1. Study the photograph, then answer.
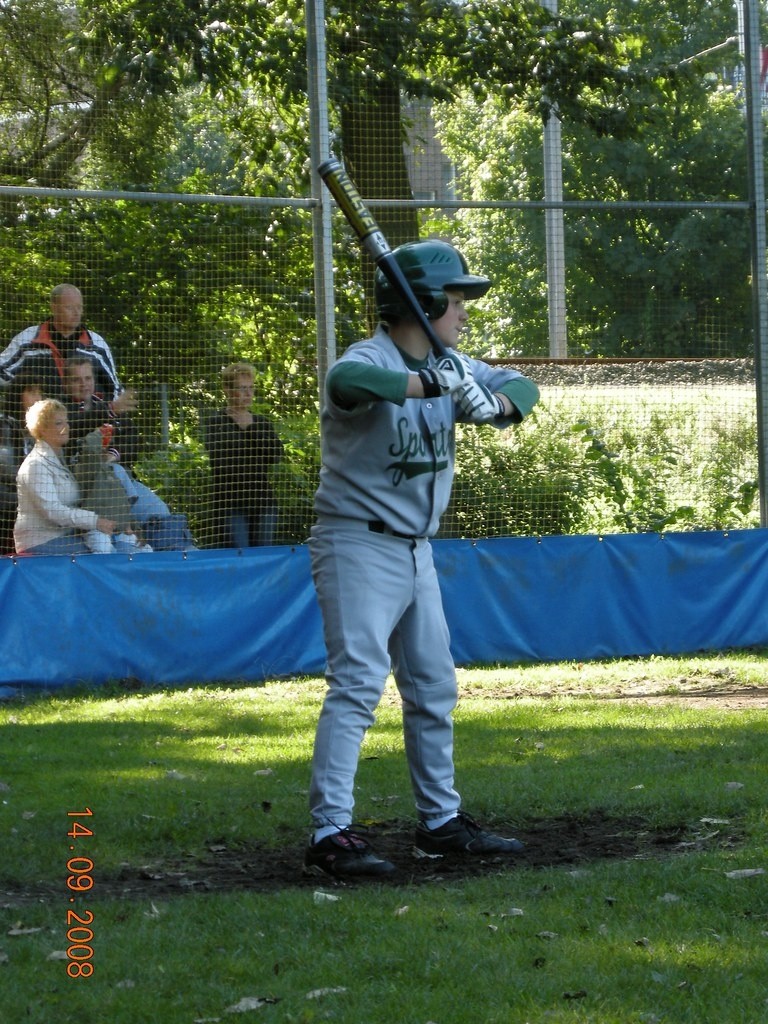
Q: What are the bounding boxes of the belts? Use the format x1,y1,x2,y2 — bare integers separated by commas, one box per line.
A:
368,519,417,540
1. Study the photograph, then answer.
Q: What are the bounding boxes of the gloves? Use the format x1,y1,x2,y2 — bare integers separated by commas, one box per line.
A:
419,356,474,398
458,381,505,420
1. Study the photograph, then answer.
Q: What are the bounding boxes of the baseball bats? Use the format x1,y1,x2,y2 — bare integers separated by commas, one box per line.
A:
316,154,454,364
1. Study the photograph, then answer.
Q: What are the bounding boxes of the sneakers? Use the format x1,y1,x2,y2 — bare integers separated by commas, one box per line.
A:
414,815,522,866
304,822,397,885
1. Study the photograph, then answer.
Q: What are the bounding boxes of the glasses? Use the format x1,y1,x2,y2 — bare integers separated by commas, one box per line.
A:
236,385,255,391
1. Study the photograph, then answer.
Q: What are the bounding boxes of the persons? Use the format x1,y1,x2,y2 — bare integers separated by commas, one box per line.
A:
0,283,125,444
9,373,47,464
64,356,138,463
203,363,285,548
13,399,153,553
302,240,540,884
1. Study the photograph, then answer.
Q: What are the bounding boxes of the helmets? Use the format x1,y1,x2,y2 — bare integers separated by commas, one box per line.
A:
376,241,491,321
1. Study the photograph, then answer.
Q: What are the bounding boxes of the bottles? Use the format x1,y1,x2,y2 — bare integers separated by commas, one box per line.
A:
99,423,113,450
